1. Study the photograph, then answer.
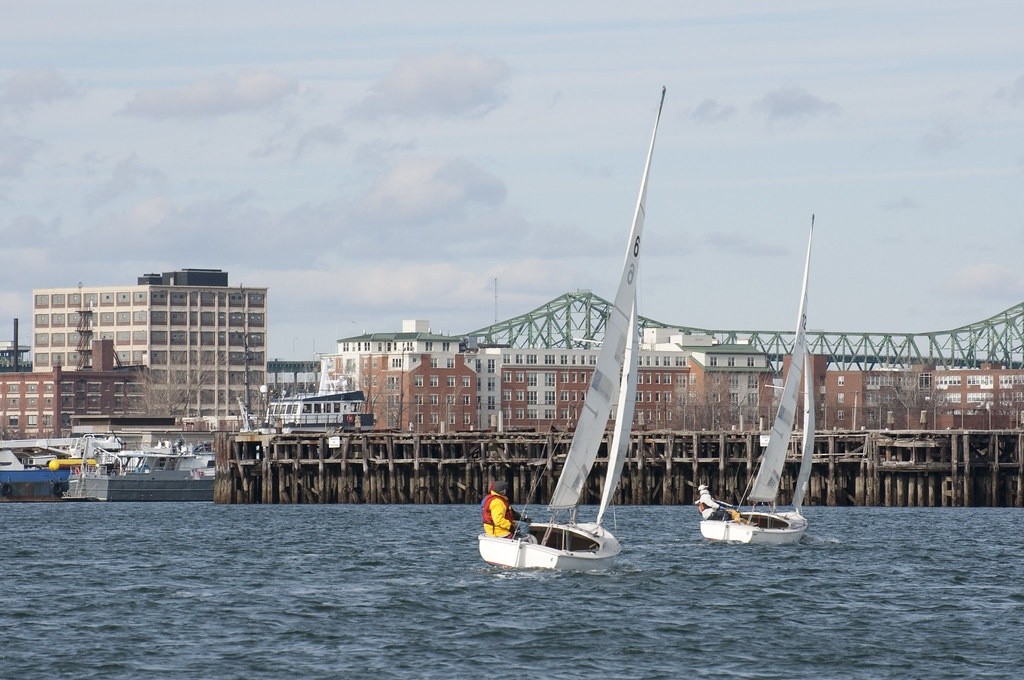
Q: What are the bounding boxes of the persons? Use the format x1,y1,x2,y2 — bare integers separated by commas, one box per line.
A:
481,481,531,540
694,485,733,521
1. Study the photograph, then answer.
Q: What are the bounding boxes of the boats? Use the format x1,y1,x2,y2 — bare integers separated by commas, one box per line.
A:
254,388,376,433
67,440,220,505
0,428,124,503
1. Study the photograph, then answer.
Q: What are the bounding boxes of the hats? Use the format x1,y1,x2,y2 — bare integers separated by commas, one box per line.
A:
698,485,708,491
695,494,711,504
493,481,509,491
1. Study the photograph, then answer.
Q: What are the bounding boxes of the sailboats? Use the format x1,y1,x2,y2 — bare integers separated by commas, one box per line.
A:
474,85,670,570
697,212,819,544
